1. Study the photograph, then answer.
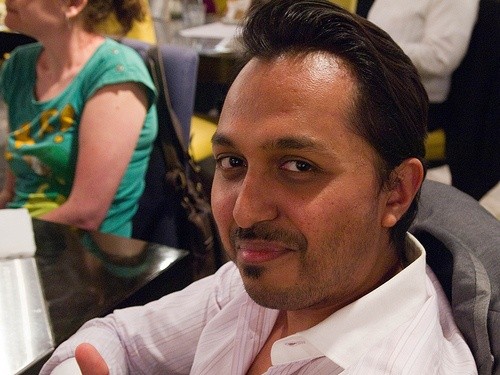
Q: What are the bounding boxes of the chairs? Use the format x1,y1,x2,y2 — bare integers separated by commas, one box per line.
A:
119,39,198,294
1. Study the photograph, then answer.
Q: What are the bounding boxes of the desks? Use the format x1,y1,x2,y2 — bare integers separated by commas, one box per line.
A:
156,15,250,119
0,217,191,375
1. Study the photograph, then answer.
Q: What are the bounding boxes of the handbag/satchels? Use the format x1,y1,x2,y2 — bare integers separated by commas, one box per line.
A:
148,46,228,283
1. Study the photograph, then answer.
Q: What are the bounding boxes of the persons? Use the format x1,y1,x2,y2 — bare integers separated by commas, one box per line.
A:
0,0,158,237
38,0,480,375
